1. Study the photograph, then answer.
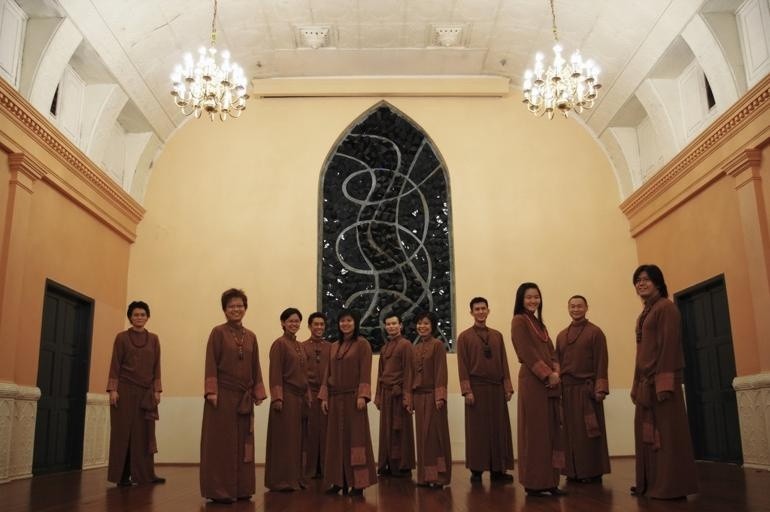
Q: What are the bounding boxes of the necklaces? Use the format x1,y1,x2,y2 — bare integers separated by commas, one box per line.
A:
566,319,589,345
309,337,324,364
128,326,150,349
473,325,493,360
336,338,354,361
227,320,246,361
635,294,667,345
524,312,549,344
383,338,398,360
295,339,306,367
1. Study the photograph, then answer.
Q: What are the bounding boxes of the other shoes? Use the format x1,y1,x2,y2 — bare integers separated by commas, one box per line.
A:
205,497,237,504
271,487,293,492
433,482,443,488
349,486,363,496
238,495,253,501
416,482,431,488
568,476,603,482
490,471,514,483
470,474,483,489
392,469,412,479
631,486,637,492
325,484,341,495
524,486,569,496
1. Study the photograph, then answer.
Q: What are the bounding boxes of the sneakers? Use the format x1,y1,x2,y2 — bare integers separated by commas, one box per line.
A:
116,475,166,487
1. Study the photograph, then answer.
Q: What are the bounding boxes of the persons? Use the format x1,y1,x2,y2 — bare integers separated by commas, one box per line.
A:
372,312,414,482
555,295,612,486
456,296,514,483
302,311,334,480
198,287,267,506
317,308,378,498
510,282,570,498
264,307,313,494
106,299,166,488
630,264,692,501
402,311,453,490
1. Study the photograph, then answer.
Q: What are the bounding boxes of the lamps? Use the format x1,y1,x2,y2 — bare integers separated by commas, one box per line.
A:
520,0,602,120
166,1,255,121
